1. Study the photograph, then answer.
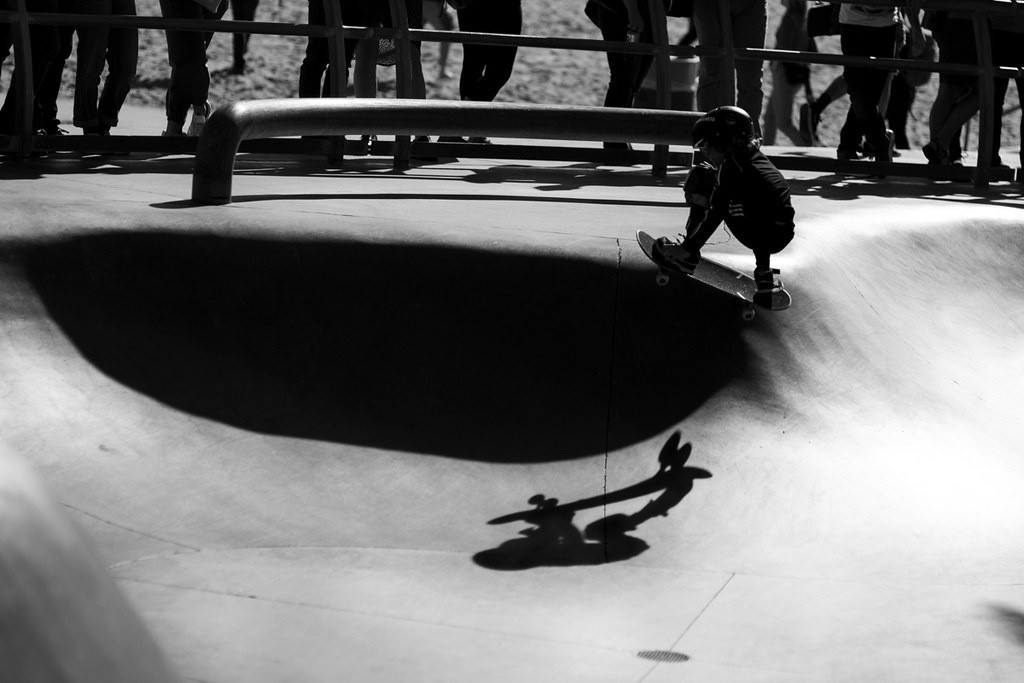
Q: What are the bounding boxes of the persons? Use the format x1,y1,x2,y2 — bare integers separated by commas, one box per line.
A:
0,0,1024,194
656,106,795,293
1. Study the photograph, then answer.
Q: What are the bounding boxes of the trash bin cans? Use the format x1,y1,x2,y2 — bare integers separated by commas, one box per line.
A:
632,54,701,114
